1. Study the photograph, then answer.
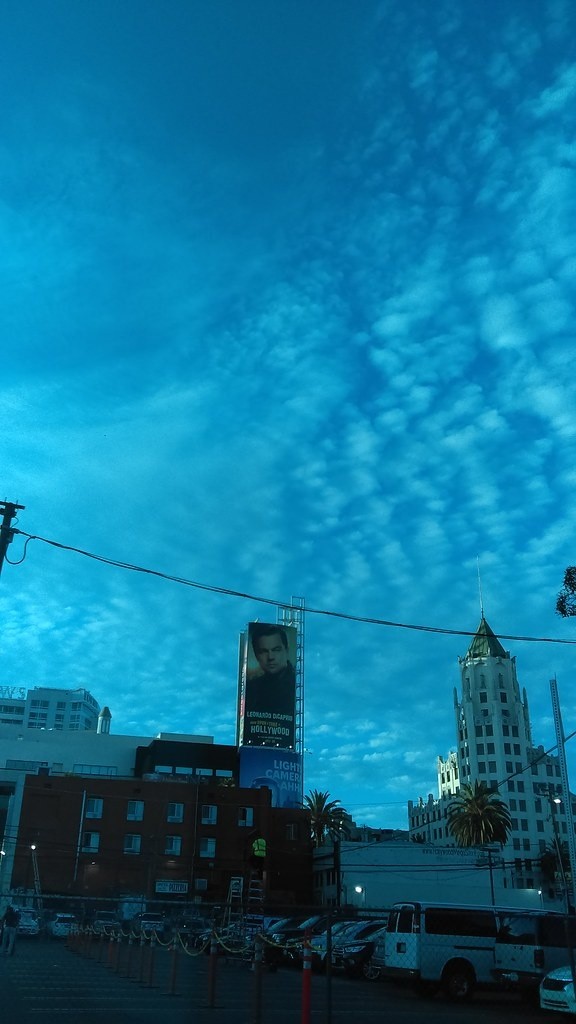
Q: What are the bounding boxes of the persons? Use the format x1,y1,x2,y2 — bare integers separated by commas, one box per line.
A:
0,905,17,957
243,622,299,749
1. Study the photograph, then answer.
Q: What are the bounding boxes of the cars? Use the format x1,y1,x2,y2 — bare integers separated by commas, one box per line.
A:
9,892,576,1015
